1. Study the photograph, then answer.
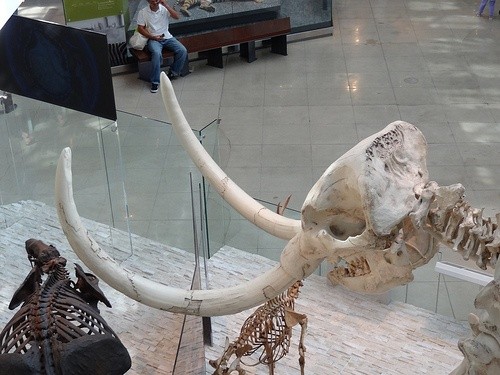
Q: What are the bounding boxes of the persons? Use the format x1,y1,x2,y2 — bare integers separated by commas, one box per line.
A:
136,0,187,93
475,0,497,20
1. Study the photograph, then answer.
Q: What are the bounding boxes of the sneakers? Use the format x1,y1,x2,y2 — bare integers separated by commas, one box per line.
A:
168,72,174,80
151,83,158,92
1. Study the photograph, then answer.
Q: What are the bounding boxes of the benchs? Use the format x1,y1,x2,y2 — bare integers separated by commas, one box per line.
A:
128,15,292,80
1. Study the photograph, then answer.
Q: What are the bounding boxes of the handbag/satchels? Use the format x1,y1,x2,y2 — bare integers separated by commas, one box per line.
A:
128,31,148,50
